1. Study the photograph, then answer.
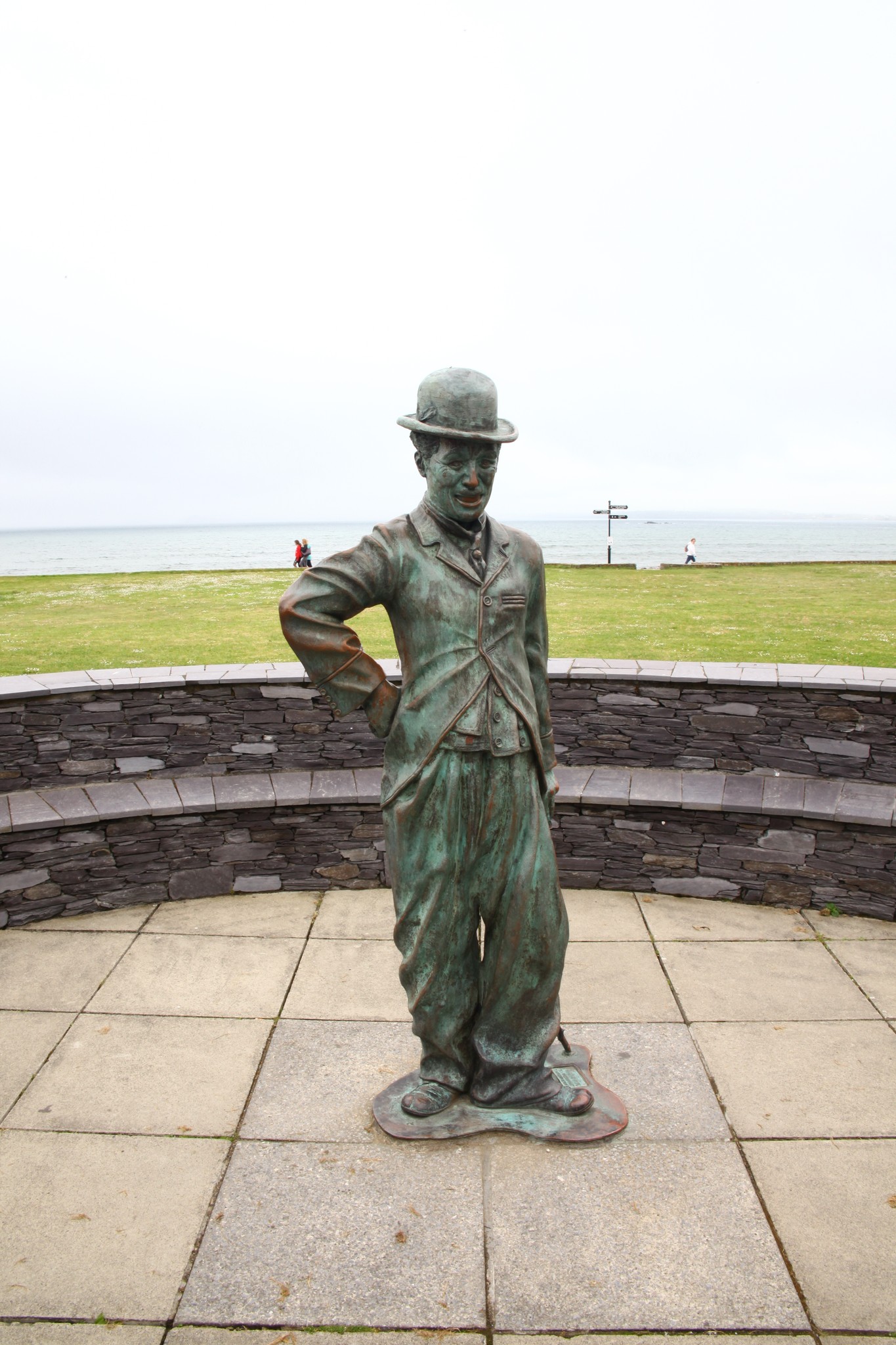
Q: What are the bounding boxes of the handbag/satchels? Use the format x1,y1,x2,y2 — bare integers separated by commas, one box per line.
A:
685,545,689,552
301,548,311,556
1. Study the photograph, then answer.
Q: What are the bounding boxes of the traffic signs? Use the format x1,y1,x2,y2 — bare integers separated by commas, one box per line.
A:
608,515,628,519
593,510,610,514
609,505,628,509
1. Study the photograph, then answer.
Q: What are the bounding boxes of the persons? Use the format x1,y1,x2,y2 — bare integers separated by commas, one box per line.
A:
276,365,600,1118
299,538,313,567
293,540,304,567
684,538,697,564
